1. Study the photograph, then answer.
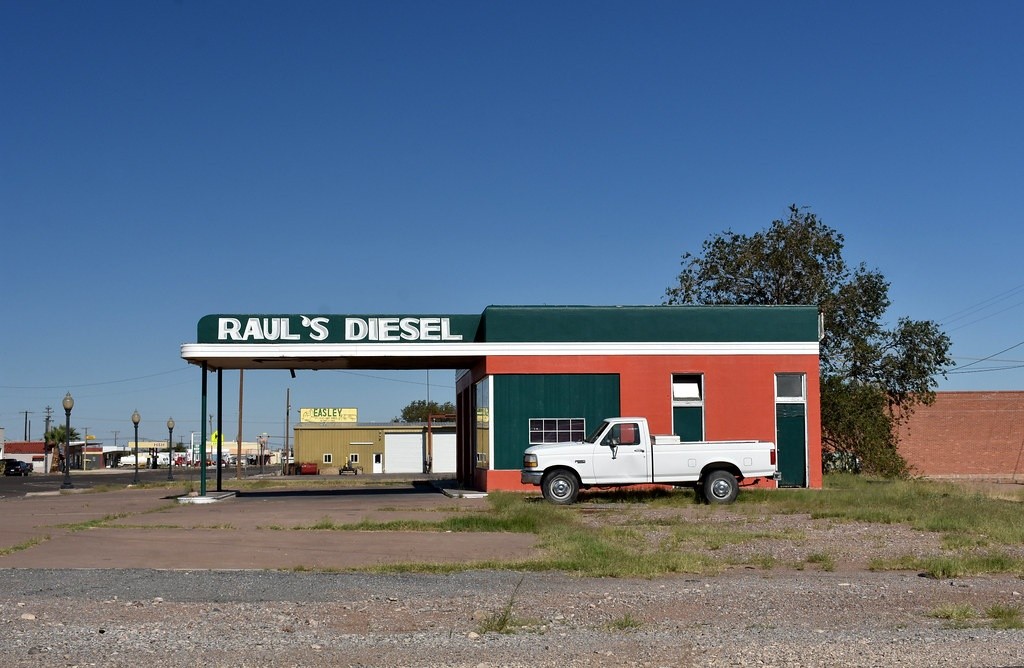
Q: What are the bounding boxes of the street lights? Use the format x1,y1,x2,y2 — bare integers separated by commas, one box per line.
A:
131,408,141,485
167,416,175,482
60,391,75,489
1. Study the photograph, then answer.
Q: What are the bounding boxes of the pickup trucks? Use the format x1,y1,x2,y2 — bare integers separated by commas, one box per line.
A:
521,417,782,507
0,459,33,472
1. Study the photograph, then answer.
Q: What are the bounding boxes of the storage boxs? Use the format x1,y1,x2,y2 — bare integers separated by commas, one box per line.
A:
650,434,680,445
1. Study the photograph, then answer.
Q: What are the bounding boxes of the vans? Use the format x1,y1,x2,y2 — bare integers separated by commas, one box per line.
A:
4,461,27,477
